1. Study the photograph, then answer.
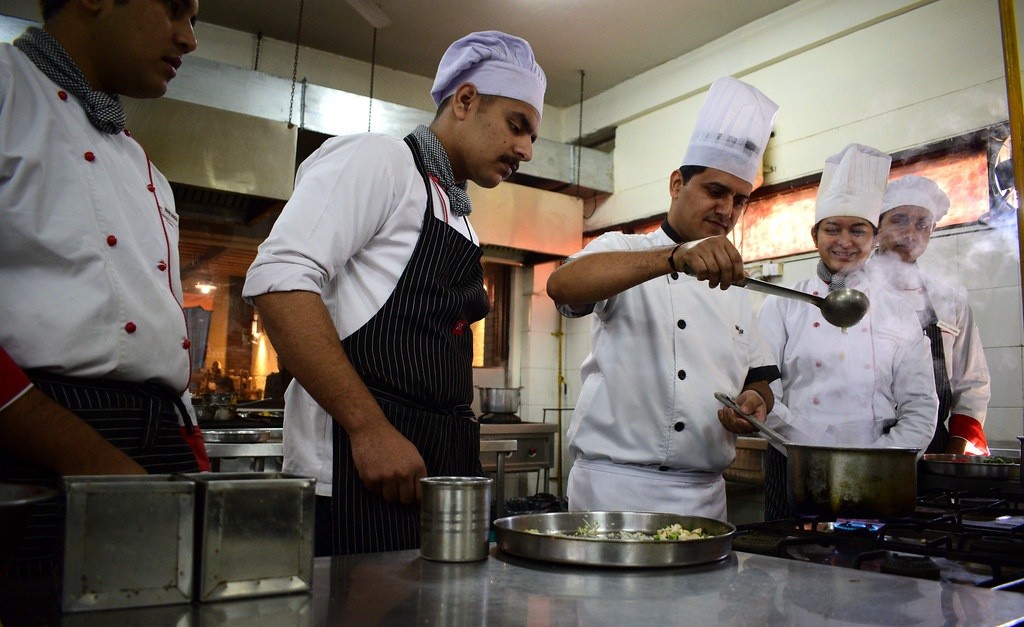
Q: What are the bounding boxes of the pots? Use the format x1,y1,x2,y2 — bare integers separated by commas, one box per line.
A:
193,397,273,422
918,454,1020,489
474,385,524,413
714,393,922,518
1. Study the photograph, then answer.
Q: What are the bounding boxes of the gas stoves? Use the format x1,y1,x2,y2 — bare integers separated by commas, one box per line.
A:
731,436,1024,591
478,413,558,471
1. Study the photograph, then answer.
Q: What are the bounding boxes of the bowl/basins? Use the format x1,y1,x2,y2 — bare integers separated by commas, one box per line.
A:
202,393,235,403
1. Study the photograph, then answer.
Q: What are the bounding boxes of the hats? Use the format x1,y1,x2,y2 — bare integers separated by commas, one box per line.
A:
880,175,951,223
429,30,547,120
814,143,892,228
681,77,779,192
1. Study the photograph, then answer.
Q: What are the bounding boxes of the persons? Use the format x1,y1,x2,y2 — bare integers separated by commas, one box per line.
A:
243,27,548,557
543,131,782,525
1,2,215,612
862,174,993,456
756,177,939,519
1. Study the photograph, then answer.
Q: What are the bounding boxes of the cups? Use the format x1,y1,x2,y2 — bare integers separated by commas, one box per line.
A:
419,477,493,561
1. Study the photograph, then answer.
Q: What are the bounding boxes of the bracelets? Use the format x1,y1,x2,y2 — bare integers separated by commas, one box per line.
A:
668,243,687,273
